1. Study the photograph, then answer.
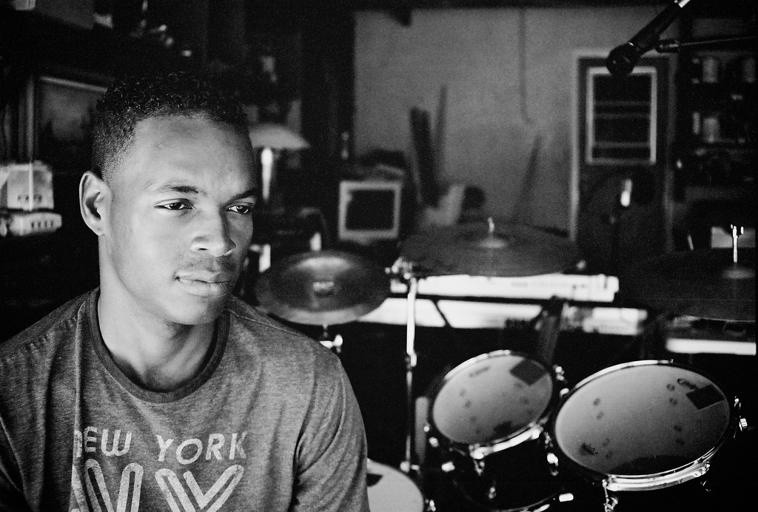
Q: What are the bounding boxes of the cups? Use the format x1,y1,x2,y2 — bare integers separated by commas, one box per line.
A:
689,52,723,145
739,55,758,83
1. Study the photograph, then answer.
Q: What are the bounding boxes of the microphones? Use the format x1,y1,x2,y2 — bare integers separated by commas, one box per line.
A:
607,0,691,80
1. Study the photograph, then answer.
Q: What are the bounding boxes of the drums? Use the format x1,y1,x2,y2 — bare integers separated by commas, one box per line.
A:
366,457,423,512
423,348,572,512
538,358,749,512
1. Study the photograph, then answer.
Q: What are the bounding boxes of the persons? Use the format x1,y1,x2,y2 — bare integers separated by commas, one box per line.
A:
0,72,369,511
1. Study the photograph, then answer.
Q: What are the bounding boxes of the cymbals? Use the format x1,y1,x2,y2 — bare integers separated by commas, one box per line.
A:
400,220,579,276
252,251,390,327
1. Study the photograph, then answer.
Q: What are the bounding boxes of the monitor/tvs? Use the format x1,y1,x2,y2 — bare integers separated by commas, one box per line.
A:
337,180,402,242
